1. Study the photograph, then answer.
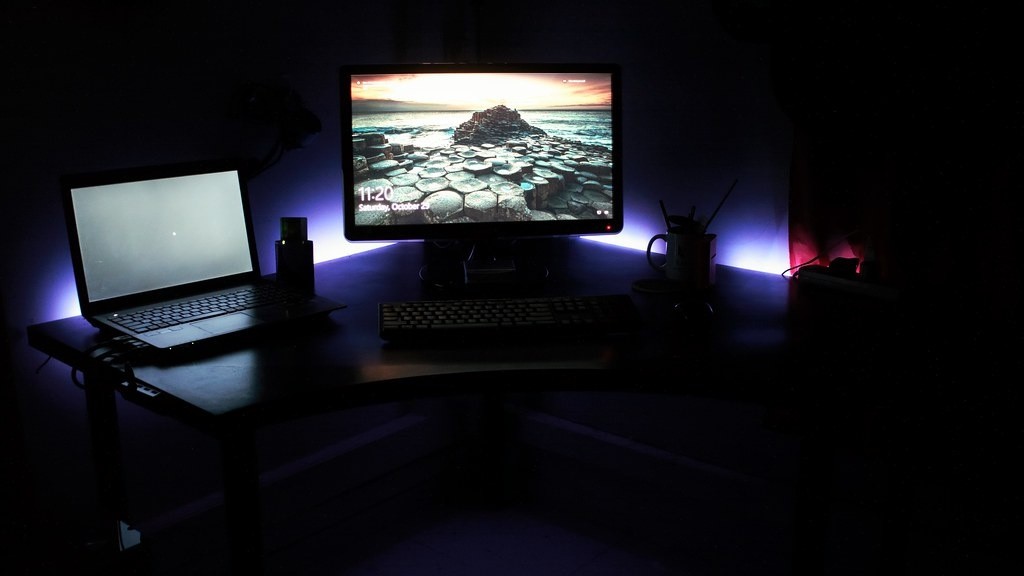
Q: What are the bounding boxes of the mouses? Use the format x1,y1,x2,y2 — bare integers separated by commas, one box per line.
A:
673,300,714,321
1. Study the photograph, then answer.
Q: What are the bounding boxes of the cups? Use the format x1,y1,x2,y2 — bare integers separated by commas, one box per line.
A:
647,228,716,288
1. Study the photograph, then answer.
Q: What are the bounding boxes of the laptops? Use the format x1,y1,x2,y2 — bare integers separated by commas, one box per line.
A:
56,158,347,359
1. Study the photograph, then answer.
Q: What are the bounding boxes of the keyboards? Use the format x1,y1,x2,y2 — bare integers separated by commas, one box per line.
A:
379,294,643,345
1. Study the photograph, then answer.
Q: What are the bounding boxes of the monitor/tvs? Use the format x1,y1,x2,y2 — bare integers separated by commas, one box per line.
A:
337,63,623,299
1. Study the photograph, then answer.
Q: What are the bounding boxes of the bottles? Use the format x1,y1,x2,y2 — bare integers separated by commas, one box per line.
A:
275,218,315,294
